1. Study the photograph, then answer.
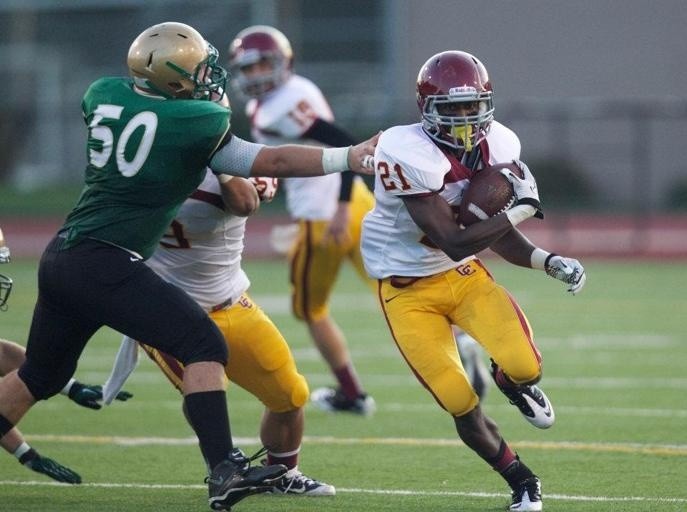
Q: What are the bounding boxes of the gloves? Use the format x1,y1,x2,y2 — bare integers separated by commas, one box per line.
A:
17,446,82,483
500,159,544,220
68,380,133,409
544,253,587,296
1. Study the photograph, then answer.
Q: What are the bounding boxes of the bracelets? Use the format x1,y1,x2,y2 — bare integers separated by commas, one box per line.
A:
505,205,537,223
217,173,238,183
528,247,549,270
320,145,351,176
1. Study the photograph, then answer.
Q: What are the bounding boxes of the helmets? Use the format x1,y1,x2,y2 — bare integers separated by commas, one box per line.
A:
227,25,292,102
416,50,496,152
0,229,14,313
127,22,227,104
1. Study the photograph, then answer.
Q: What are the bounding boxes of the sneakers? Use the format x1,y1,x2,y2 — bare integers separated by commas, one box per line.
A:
311,388,376,418
488,357,554,430
509,476,542,512
260,460,336,496
455,332,485,404
203,446,289,511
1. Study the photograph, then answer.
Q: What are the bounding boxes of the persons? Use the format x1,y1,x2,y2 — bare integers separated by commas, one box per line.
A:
1,228,135,483
360,50,587,511
227,26,492,419
141,79,340,499
1,22,384,510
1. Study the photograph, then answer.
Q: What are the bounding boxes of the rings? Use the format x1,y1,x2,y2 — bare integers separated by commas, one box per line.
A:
360,156,370,170
368,157,373,169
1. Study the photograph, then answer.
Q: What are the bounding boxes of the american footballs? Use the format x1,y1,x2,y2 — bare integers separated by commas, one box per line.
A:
459,162,525,226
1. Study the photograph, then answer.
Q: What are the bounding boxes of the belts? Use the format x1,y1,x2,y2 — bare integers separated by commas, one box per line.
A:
389,275,421,288
